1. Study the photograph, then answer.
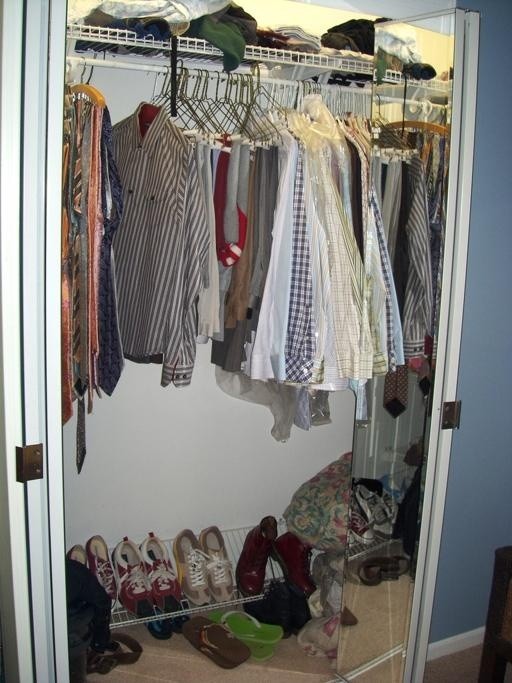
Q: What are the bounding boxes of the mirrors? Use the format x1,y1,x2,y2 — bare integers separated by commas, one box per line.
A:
342,6,459,681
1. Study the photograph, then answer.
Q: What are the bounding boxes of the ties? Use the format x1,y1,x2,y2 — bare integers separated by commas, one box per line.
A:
61,97,124,476
383,130,450,420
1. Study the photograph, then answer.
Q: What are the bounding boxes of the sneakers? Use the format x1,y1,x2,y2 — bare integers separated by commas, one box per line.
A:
355,473,419,538
350,510,376,546
114,538,154,616
142,536,182,606
88,535,119,610
68,543,86,565
173,528,211,606
200,526,234,603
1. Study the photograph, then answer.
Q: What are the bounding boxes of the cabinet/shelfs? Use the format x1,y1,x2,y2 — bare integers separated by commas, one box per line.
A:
372,94,452,159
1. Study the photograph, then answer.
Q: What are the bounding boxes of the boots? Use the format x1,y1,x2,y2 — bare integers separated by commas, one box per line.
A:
234,516,277,597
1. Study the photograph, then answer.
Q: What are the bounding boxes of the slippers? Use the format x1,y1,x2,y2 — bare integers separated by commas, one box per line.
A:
182,609,283,670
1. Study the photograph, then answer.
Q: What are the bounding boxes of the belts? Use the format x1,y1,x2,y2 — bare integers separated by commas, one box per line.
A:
357,555,411,588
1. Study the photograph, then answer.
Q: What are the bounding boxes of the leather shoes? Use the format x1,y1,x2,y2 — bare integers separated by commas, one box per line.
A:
242,582,313,638
146,602,183,641
270,532,317,600
